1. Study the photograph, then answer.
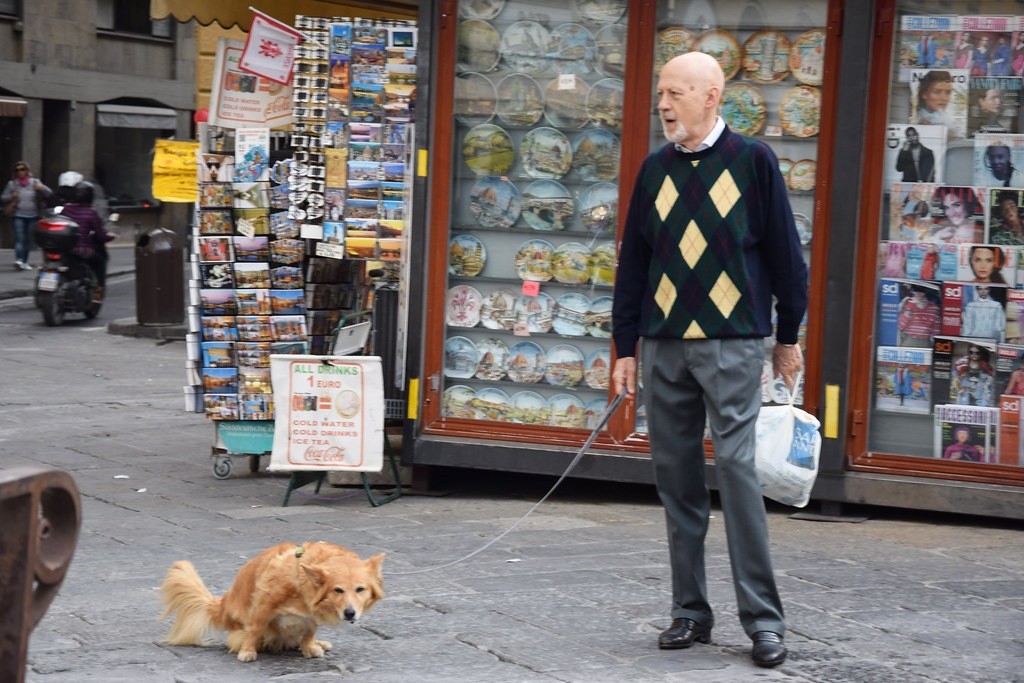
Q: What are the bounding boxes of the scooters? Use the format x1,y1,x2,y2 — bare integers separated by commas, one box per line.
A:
35,184,119,326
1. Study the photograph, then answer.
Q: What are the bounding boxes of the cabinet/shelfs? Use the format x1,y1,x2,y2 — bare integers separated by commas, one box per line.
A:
419,0,844,460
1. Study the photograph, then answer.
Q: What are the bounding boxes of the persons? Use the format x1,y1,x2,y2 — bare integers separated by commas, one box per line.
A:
1011,32,1024,77
991,190,1024,245
45,170,116,304
971,35,990,76
894,365,912,406
967,82,1007,139
962,286,1005,341
917,32,934,67
958,364,994,406
895,127,934,183
982,141,1023,189
914,71,955,137
954,31,973,69
898,286,940,347
991,36,1011,76
931,187,984,243
610,52,807,667
969,246,1005,284
919,243,940,279
956,344,993,376
0,161,53,269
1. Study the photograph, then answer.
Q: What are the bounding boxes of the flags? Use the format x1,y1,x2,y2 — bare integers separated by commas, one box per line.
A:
238,13,299,87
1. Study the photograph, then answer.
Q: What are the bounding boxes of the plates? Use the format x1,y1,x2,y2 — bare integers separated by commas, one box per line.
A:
792,213,813,246
447,285,483,327
778,86,822,138
515,292,555,334
586,296,614,339
777,158,817,190
460,124,621,181
480,287,518,331
552,293,590,337
454,72,625,132
652,25,827,84
442,385,651,440
468,176,619,238
721,82,767,135
570,0,627,28
759,359,805,405
443,335,612,391
457,20,627,78
456,0,507,20
447,235,487,277
513,238,617,287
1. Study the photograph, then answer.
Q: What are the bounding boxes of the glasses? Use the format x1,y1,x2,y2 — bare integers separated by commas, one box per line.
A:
15,168,27,171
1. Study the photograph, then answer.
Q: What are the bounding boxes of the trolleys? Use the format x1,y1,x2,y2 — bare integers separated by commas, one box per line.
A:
211,311,374,481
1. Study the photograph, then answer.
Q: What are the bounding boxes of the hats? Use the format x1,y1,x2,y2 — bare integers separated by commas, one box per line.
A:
57,171,84,186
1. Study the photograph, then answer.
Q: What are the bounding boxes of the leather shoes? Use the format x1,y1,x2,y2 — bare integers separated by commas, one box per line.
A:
658,617,711,649
752,631,788,667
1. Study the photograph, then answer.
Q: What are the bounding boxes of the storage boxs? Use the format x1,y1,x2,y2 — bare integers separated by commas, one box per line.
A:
33,215,80,252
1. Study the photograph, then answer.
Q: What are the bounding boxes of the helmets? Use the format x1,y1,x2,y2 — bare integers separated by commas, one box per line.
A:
72,180,95,206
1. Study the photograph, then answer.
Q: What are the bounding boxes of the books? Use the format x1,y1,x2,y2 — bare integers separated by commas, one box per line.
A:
197,126,308,420
873,14,1024,468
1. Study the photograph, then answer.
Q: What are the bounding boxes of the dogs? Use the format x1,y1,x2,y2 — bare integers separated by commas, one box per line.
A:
156,540,385,662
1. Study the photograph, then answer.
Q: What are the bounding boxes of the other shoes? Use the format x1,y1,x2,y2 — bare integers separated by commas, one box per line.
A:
21,263,33,271
12,260,24,270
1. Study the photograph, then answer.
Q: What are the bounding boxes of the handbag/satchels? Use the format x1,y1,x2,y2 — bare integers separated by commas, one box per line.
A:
2,182,20,218
750,366,822,508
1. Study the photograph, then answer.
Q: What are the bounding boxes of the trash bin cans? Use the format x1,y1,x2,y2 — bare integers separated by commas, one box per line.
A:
135,228,184,325
